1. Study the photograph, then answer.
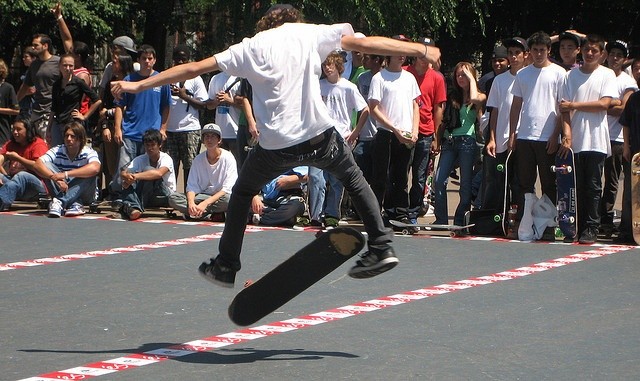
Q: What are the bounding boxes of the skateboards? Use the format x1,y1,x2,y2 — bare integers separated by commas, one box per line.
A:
419,151,435,217
389,220,475,237
631,152,640,245
494,150,513,236
159,207,177,218
228,226,366,326
550,148,577,238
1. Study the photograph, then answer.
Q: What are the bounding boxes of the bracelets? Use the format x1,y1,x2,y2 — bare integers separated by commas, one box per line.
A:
562,136,571,144
102,120,108,129
420,45,428,59
56,15,62,22
187,95,193,103
105,107,115,117
65,171,68,179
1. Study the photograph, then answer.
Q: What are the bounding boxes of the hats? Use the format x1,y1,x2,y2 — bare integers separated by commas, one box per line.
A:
24,46,38,59
113,36,138,54
503,37,529,53
490,46,508,59
391,35,412,43
202,123,222,137
418,38,435,47
559,32,581,48
605,39,630,59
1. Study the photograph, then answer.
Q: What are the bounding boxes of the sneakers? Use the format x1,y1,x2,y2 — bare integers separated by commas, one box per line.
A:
347,242,398,279
197,254,236,288
507,224,520,239
425,223,448,231
292,219,323,230
323,217,339,232
129,204,141,221
410,218,420,233
65,202,86,217
579,227,599,245
212,211,227,223
48,197,63,218
393,218,418,233
541,226,556,241
564,233,579,243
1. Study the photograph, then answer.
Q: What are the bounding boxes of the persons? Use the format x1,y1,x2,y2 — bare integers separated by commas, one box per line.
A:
469,41,510,209
16,33,61,145
50,54,102,148
558,32,616,243
108,3,441,289
423,61,487,231
33,121,102,217
0,60,21,149
597,37,638,237
399,35,448,234
98,35,141,106
346,51,371,151
206,44,241,164
169,123,238,221
338,54,386,224
246,169,309,228
113,43,172,175
485,37,530,234
307,50,370,227
164,45,210,193
98,54,136,210
631,54,640,81
549,29,587,71
507,30,567,240
15,45,38,119
233,76,260,171
49,0,92,115
0,116,51,212
366,34,422,228
618,90,640,245
118,128,176,220
